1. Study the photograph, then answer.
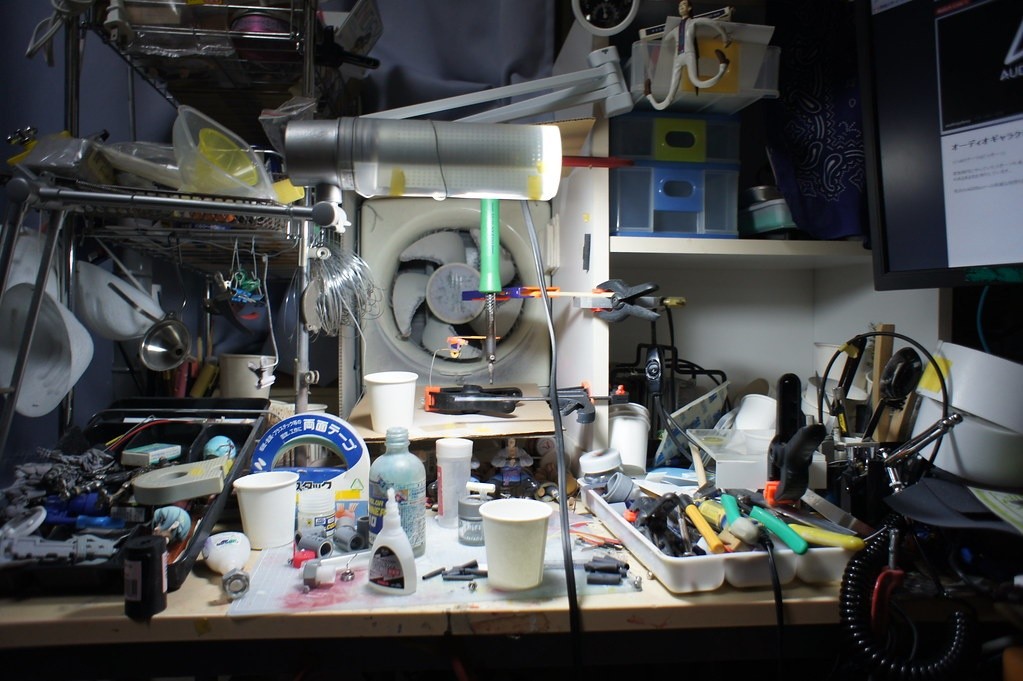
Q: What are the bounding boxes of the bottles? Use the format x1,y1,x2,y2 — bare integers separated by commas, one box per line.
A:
436,438,473,528
580,448,625,489
201,531,251,600
367,487,416,594
297,488,336,538
369,427,426,558
458,494,493,546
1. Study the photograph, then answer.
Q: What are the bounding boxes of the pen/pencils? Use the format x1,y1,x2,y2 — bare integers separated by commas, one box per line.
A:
164,363,199,397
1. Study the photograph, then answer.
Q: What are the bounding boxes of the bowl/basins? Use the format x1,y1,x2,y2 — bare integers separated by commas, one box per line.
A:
171,104,274,199
195,127,259,190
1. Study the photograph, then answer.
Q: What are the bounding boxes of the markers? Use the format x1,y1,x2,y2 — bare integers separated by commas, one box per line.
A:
189,364,220,397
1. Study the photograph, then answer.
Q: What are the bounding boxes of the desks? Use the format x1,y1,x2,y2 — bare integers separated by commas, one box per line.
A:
0,474,895,681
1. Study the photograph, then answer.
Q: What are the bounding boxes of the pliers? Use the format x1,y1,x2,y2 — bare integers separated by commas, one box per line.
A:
717,486,807,554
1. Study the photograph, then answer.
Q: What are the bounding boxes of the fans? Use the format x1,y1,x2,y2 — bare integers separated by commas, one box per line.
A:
359,195,551,409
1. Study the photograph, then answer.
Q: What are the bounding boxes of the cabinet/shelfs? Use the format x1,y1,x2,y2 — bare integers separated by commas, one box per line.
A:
38,0,382,515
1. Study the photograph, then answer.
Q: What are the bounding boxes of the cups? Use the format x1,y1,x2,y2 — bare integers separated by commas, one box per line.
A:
478,497,554,590
306,403,328,413
364,371,419,435
800,376,868,438
606,402,652,475
233,471,299,550
809,342,869,392
217,352,276,400
123,534,169,619
722,394,778,452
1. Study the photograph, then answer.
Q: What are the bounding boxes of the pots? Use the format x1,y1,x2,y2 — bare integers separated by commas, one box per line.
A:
229,11,380,70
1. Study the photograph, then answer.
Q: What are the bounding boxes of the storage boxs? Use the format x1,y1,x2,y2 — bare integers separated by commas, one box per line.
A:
609,112,740,238
